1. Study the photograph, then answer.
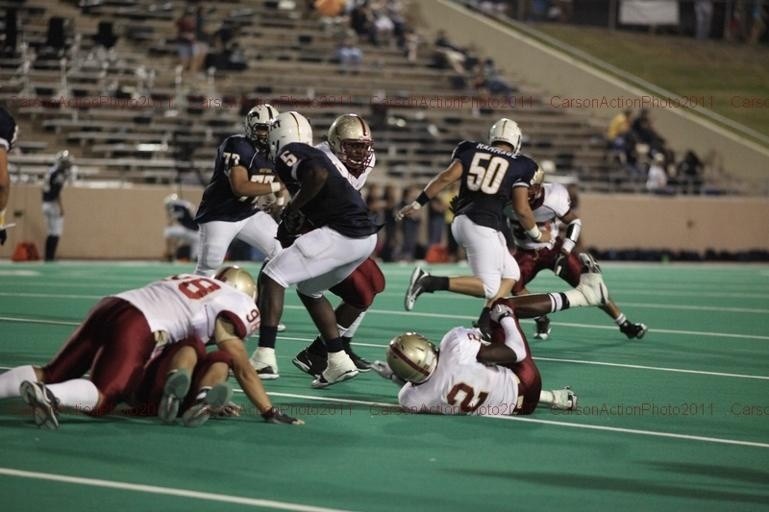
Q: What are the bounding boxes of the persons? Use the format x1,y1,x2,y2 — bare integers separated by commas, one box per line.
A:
40,149,75,262
369,251,611,419
290,112,386,380
192,103,287,332
504,163,648,339
162,194,201,263
0,262,306,431
394,117,553,344
0,106,20,247
123,335,244,428
227,110,387,389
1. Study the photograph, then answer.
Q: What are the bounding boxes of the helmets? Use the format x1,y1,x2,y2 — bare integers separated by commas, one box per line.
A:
387,330,437,385
55,150,77,168
489,117,521,158
214,264,257,303
513,161,546,204
245,103,280,149
267,111,313,162
327,113,375,177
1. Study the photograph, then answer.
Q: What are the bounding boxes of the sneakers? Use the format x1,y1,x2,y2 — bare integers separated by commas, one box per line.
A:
534,316,553,339
293,336,326,380
158,369,191,423
552,385,577,411
312,350,359,388
225,348,278,379
621,319,649,340
20,380,58,431
577,251,609,305
348,351,373,373
403,267,431,311
183,382,235,428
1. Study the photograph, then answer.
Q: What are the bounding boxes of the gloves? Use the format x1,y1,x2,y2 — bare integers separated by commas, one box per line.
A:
274,212,300,249
490,298,515,323
370,362,392,379
261,407,296,425
